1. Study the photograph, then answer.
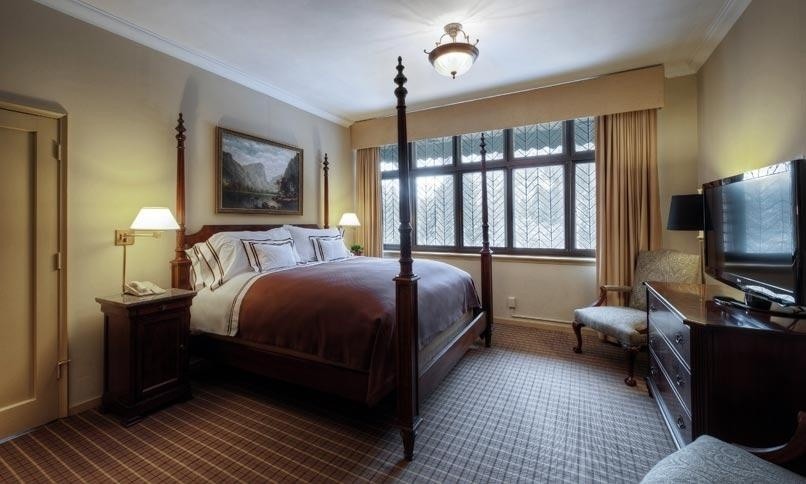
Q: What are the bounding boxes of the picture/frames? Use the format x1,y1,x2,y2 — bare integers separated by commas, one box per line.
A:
211,126,304,216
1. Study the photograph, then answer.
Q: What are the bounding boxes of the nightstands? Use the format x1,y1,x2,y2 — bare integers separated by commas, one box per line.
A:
95,287,198,428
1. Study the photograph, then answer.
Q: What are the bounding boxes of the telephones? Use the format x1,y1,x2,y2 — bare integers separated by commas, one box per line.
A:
125,279,165,296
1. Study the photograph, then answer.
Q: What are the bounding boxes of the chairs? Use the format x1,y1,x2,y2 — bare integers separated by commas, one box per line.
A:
639,411,805,484
572,248,703,386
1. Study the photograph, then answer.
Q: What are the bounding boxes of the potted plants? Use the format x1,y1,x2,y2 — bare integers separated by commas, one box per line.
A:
349,243,364,256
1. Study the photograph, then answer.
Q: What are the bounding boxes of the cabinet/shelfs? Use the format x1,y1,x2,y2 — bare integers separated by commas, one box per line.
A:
641,279,806,451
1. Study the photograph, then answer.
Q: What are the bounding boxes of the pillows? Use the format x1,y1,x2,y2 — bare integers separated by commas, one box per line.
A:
184,225,354,292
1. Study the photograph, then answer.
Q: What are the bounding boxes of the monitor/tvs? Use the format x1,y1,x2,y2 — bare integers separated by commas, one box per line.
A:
698,156,806,319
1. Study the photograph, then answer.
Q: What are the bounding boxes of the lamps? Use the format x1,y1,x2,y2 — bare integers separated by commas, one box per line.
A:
337,212,361,232
423,22,480,80
667,193,713,256
115,207,181,245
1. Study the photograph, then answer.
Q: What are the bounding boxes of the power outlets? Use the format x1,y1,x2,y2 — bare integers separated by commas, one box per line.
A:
508,296,515,308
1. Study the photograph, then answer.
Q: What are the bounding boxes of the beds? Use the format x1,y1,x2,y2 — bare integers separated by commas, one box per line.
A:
169,54,494,462
